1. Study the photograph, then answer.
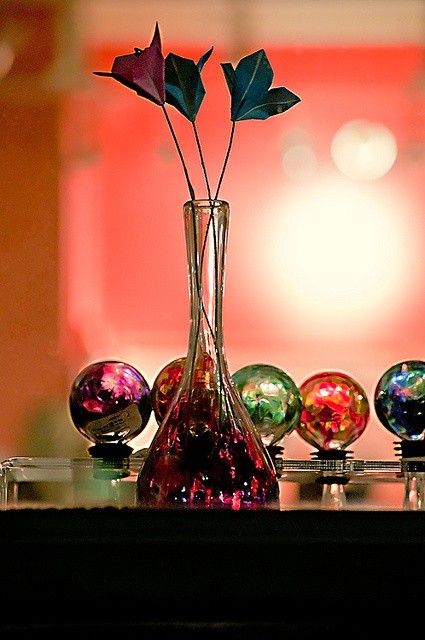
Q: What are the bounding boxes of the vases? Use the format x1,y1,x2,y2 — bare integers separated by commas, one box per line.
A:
136,198,281,510
